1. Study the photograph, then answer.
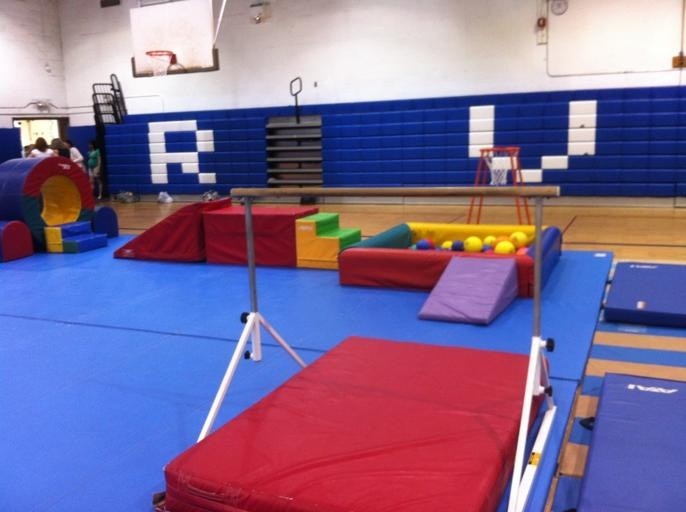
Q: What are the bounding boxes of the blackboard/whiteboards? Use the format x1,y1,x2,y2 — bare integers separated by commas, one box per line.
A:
546,0,684,77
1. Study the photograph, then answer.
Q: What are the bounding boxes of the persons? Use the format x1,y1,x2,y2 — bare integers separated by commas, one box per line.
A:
24,138,102,200
